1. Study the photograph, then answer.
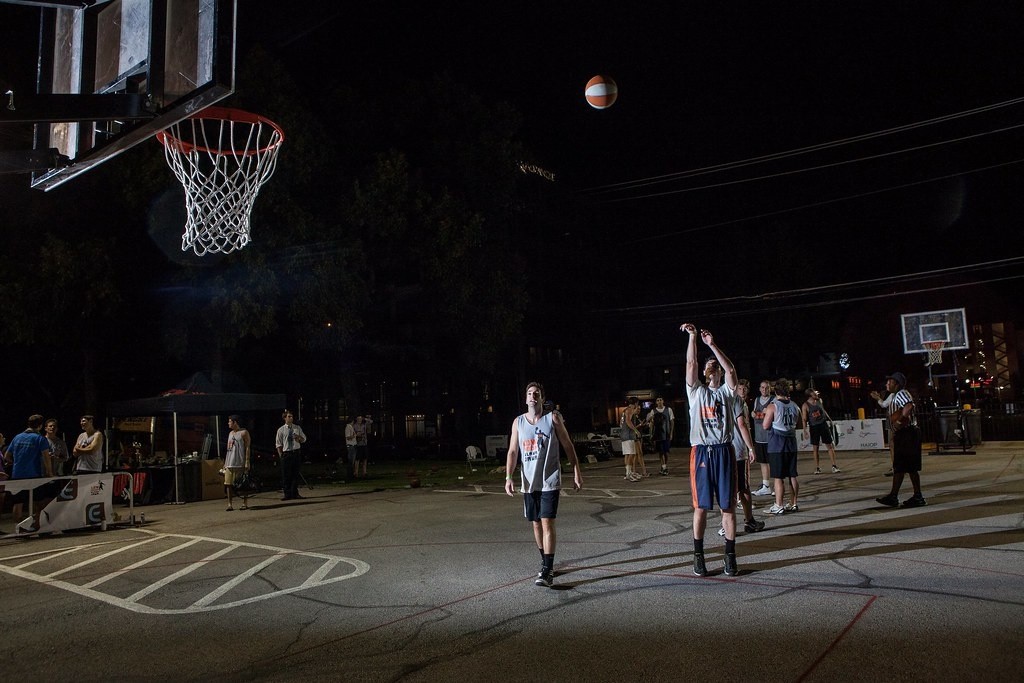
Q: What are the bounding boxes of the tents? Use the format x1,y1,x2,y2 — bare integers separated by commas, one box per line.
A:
102,384,293,505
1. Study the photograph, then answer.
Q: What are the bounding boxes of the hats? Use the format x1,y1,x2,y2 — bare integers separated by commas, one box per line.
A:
886,372,906,388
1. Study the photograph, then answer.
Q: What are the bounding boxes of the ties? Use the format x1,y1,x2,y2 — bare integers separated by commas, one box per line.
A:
287,426,294,451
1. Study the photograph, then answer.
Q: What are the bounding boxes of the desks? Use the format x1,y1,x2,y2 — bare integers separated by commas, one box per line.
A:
107,460,202,507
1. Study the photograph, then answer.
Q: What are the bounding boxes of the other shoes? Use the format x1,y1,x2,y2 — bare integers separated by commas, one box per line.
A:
814,467,822,475
903,497,925,506
280,497,291,501
882,467,894,477
642,472,652,478
718,528,725,536
832,465,841,473
876,494,899,507
624,472,641,482
658,468,670,476
292,495,307,499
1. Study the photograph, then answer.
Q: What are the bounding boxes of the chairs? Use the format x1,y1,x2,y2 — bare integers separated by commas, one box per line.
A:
465,446,487,474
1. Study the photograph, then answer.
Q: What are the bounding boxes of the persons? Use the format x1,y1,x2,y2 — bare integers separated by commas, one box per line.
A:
620,396,674,482
718,371,927,537
276,410,307,500
680,323,740,576
224,414,250,511
505,382,584,587
345,416,369,481
0,414,127,538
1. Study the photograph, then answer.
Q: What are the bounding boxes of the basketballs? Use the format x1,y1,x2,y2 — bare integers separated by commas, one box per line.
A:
410,479,420,488
891,410,909,428
584,75,618,110
431,465,438,472
408,467,416,475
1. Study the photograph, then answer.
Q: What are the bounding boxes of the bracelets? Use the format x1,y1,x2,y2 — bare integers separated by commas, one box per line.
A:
749,448,755,451
897,420,902,424
506,475,512,480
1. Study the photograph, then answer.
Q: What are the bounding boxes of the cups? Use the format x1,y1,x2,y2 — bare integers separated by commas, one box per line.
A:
193,452,197,457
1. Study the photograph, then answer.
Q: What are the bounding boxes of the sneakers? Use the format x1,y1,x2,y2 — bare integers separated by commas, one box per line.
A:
783,503,798,513
751,483,776,496
763,502,784,515
535,566,554,587
723,552,739,575
537,560,545,578
694,551,707,577
744,516,766,533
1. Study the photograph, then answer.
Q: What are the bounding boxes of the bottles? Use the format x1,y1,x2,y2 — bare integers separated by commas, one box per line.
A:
102,518,106,530
141,512,145,523
82,440,88,446
